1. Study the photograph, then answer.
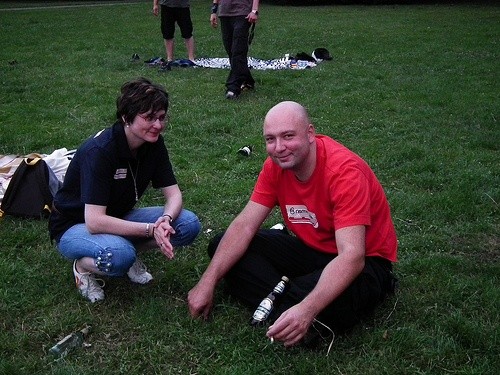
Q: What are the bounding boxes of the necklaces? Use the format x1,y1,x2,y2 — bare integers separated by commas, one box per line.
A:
128,160,138,201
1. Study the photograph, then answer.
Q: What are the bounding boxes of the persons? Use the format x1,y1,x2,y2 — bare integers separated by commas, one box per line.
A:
190,101,398,348
49,77,201,304
152,0,195,62
210,0,259,99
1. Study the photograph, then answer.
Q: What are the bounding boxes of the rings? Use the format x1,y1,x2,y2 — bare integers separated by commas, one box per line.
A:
160,243,163,246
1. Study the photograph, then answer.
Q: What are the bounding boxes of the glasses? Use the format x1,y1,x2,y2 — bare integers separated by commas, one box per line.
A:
137,113,167,123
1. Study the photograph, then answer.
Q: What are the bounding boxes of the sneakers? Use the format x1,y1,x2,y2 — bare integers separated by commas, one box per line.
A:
126,260,154,286
73,260,106,302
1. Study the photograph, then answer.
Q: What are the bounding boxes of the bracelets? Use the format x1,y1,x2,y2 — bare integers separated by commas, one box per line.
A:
152,226,155,239
146,222,150,240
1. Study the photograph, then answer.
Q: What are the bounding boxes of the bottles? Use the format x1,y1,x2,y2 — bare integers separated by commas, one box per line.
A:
248,275,290,326
237,144,253,157
48,324,93,359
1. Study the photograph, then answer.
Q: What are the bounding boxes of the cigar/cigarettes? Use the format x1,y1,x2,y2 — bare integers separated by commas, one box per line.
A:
269,336,274,343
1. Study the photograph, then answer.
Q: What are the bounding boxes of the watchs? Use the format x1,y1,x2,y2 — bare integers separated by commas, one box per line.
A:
161,215,173,225
252,9,258,15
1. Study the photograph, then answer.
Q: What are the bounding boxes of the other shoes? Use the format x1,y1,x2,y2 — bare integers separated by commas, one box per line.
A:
240,82,252,91
227,91,237,101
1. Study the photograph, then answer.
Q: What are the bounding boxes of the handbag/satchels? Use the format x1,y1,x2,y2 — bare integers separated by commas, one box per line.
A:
0,153,53,219
